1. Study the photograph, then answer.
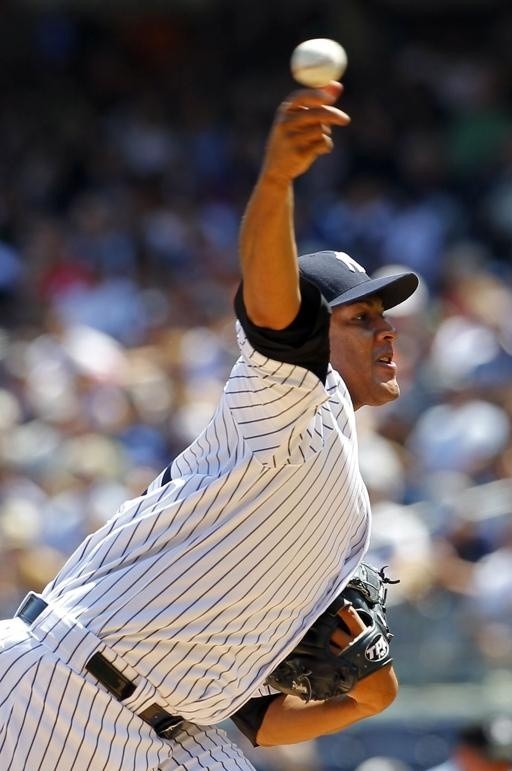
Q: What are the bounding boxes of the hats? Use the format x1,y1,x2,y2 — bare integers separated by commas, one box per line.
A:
296,249,420,309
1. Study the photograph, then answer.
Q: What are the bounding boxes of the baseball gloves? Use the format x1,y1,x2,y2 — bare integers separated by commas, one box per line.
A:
266,564,392,700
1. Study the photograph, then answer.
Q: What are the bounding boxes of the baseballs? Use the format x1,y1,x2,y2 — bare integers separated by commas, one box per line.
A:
293,39,346,85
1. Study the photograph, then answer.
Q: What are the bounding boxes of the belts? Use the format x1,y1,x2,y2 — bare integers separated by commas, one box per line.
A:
17,590,189,743
